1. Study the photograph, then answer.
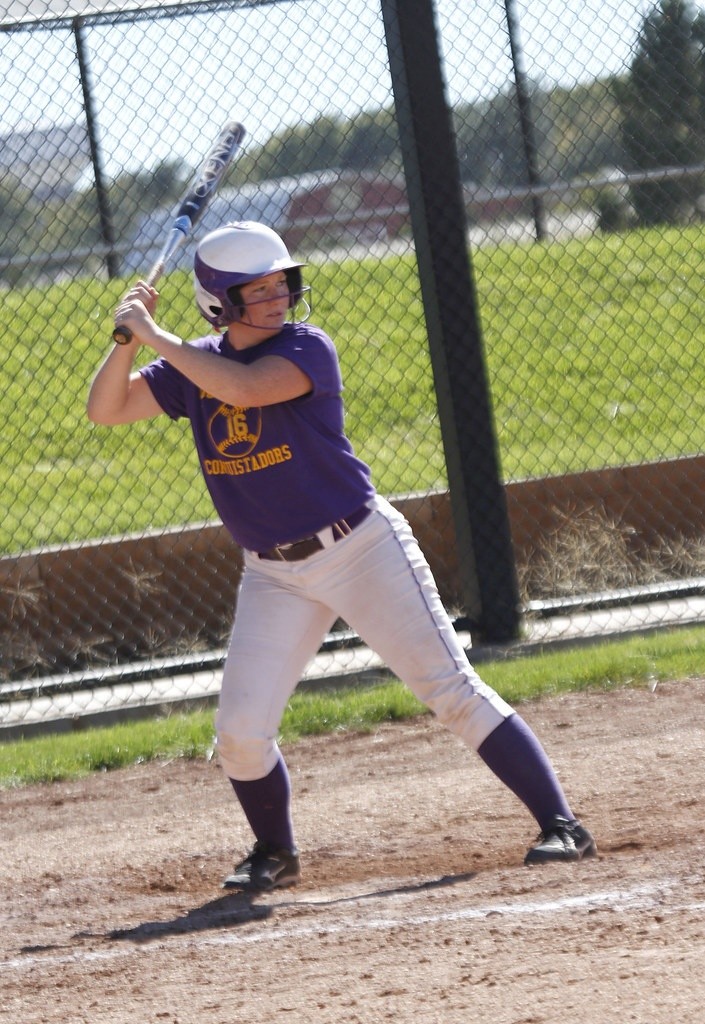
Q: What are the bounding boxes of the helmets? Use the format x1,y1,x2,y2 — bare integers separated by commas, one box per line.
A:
193,220,308,326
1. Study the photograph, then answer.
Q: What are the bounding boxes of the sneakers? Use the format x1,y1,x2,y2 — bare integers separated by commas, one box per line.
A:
225,844,302,891
523,820,599,864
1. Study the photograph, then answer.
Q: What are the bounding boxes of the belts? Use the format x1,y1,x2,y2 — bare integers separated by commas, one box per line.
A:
258,505,370,563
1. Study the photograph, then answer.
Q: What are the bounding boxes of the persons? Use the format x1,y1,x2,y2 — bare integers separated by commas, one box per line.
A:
84,222,598,893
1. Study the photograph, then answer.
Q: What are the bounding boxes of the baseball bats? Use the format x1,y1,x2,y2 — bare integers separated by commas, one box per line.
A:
113,118,247,347
